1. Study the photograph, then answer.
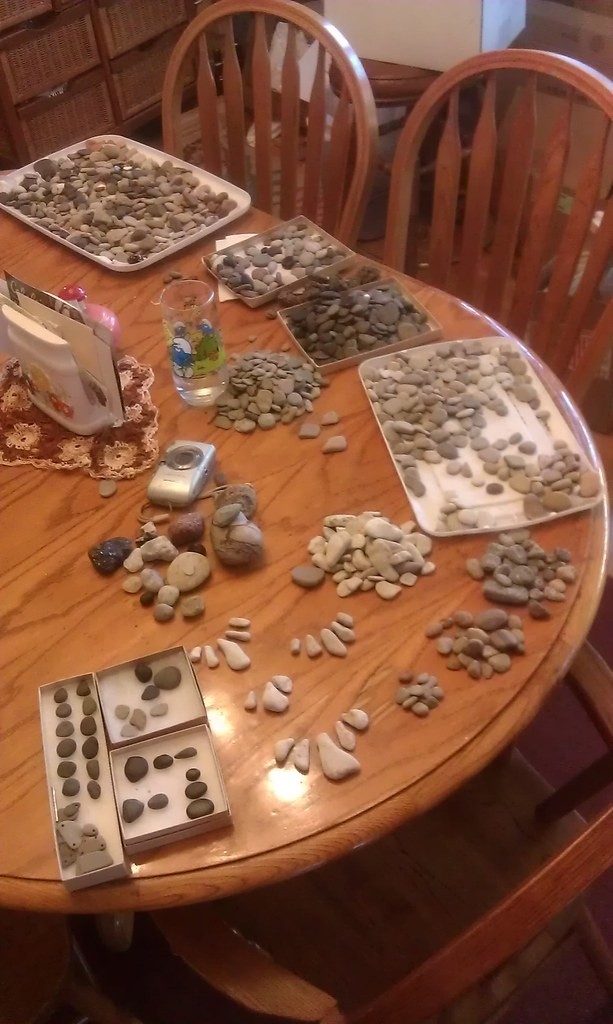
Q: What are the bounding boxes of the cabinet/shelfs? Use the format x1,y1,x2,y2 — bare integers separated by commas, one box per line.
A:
1,0,213,164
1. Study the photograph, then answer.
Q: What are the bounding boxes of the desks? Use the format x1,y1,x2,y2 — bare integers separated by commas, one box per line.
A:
0,174,611,967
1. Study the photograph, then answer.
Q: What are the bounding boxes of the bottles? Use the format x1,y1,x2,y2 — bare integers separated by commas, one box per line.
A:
59,284,120,350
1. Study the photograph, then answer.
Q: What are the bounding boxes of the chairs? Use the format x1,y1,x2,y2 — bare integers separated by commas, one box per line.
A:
384,48,613,413
163,0,377,253
105,637,613,1024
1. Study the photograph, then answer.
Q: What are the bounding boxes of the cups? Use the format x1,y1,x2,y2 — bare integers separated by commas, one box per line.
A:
160,279,230,407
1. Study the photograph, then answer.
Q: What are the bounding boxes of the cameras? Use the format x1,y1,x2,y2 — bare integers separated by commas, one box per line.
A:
146,439,216,508
